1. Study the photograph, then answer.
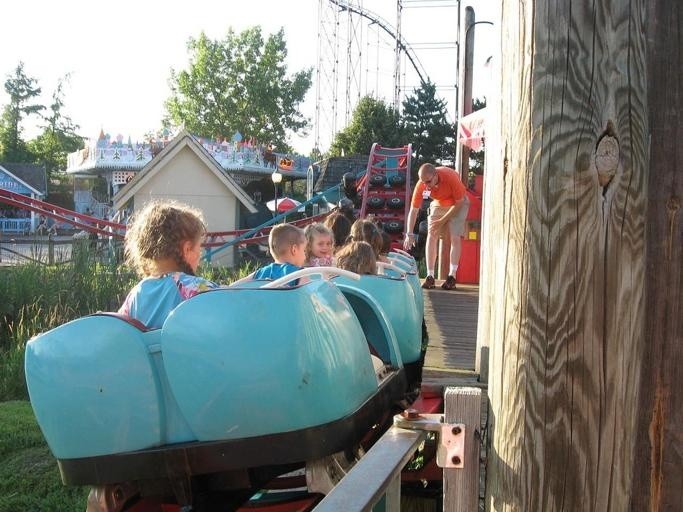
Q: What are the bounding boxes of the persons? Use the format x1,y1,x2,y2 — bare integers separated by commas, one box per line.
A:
402,162,470,290
115,201,218,329
0,201,131,241
225,211,414,287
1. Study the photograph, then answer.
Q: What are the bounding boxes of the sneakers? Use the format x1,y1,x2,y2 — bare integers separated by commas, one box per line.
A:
422,275,435,288
442,276,456,289
